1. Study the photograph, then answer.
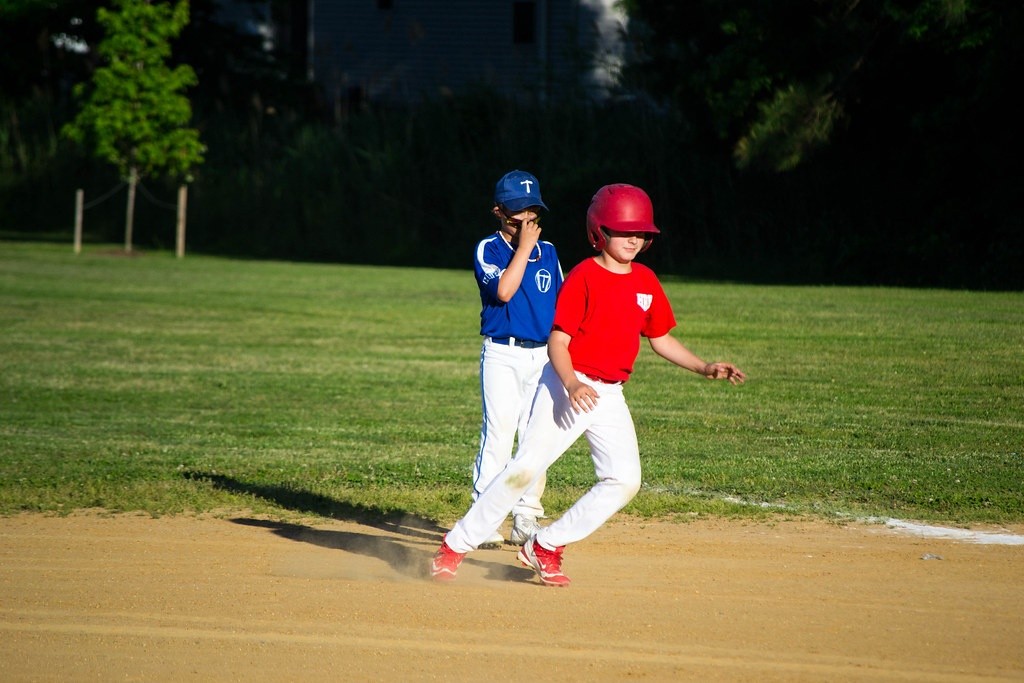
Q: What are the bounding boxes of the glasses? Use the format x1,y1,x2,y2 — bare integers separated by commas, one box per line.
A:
498,204,541,230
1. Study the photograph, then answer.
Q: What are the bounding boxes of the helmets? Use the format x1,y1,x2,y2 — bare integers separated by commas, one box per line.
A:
586,183,661,253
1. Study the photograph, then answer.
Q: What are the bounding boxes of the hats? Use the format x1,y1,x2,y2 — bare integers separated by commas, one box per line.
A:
496,170,550,212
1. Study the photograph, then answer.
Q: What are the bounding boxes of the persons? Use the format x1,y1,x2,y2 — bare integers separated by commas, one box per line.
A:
429,184,746,587
471,170,566,549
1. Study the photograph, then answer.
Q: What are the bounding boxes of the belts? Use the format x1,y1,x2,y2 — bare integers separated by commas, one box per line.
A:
586,374,619,384
492,336,547,348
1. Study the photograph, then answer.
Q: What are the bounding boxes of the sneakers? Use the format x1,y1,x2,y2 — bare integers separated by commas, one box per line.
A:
430,533,467,583
511,515,550,547
516,535,571,587
477,531,504,550
